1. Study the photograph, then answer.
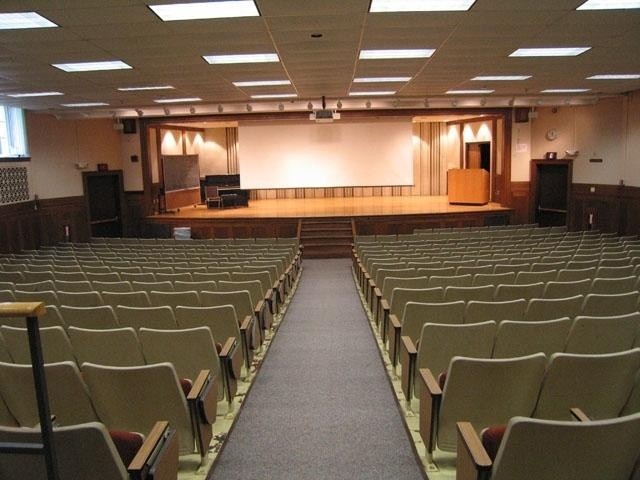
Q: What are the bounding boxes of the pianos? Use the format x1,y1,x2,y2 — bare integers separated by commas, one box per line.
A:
205,175,250,208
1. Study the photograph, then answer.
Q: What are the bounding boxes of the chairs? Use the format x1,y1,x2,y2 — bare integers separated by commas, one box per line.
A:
204,185,221,208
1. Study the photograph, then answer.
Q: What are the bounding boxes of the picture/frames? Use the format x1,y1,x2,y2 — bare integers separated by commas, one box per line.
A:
546,128,557,141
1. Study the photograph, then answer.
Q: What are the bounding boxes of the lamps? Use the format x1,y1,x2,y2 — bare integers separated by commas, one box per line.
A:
48,91,601,122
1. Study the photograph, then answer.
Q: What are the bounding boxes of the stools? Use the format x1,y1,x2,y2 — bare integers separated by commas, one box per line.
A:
221,194,238,208
